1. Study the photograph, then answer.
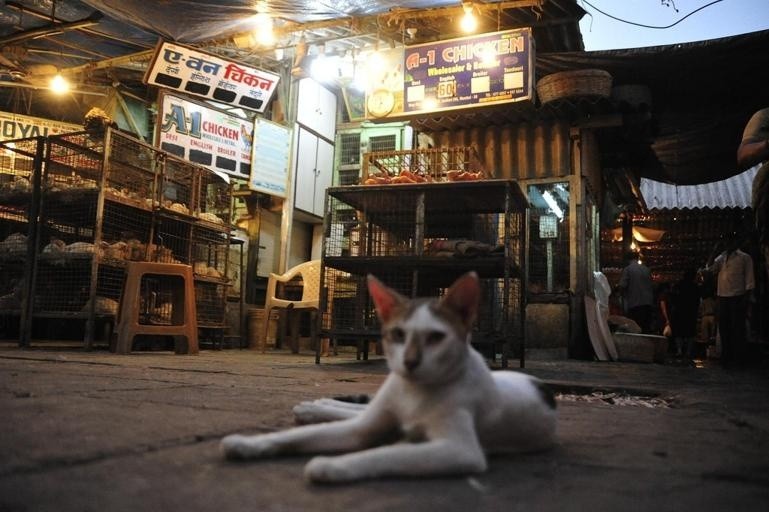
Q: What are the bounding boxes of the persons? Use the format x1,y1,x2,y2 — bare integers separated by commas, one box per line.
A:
701,289,716,338
735,98,769,210
665,267,703,336
713,230,756,368
616,250,654,336
647,266,671,337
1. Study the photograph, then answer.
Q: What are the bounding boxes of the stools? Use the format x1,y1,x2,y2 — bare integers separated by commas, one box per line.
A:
109,261,199,355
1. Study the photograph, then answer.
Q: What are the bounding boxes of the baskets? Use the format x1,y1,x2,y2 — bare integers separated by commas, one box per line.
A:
533,68,614,111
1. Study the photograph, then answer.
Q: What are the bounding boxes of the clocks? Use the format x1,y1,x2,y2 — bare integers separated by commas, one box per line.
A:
367,88,395,118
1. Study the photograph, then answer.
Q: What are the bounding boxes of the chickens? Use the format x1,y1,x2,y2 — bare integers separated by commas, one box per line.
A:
0,173,225,321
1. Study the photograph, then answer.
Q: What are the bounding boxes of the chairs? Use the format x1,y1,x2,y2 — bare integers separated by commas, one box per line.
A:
261,259,357,357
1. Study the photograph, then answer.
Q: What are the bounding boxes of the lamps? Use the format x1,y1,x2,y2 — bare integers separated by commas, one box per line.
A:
461,4,478,33
49,30,69,94
541,188,563,219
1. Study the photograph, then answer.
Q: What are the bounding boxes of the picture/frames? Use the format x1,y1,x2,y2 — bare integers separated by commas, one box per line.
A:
248,116,293,199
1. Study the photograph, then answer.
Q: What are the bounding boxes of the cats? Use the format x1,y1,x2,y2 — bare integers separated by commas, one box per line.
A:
217,270,560,486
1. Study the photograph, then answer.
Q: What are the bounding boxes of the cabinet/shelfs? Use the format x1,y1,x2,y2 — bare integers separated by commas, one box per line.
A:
0,124,237,349
272,76,338,144
268,125,335,224
316,179,530,370
